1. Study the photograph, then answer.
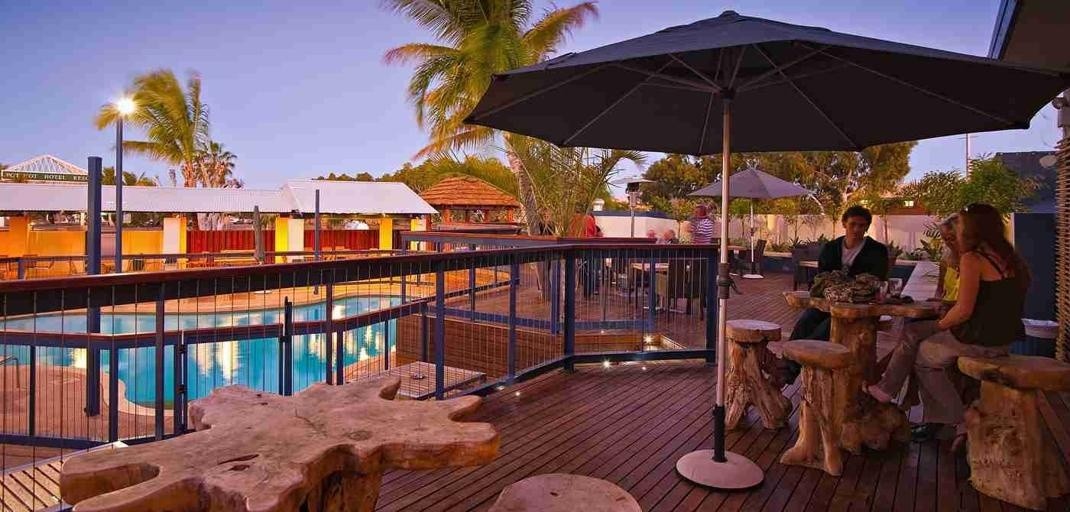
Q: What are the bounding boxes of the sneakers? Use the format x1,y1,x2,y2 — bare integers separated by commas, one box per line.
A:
770,377,784,387
756,345,797,384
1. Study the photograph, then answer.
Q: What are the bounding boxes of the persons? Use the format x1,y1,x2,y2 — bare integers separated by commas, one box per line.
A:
692,204,714,244
876,212,964,414
778,205,889,387
646,228,656,238
859,200,1034,459
663,229,679,245
529,200,603,302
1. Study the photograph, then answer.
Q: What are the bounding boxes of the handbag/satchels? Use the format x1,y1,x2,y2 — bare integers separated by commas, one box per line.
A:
809,271,849,298
823,282,877,303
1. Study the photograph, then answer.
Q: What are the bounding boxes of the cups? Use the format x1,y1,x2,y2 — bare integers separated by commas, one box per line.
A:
873,281,888,304
889,278,902,299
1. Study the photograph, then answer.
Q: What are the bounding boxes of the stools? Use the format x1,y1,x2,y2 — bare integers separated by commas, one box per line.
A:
781,338,850,476
726,319,793,428
488,474,643,512
959,354,1070,511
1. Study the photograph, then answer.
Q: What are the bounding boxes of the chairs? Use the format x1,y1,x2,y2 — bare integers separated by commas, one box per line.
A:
656,257,707,322
739,237,767,278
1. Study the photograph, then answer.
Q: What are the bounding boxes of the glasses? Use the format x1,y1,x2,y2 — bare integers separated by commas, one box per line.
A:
962,203,972,214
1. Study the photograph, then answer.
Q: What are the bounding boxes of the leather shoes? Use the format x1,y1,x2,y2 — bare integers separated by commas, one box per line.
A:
909,425,939,442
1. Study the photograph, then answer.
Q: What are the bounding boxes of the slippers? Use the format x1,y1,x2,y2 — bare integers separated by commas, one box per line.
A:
952,436,968,457
860,380,889,407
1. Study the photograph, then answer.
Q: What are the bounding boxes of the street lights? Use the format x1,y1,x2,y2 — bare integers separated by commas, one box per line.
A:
115,99,132,275
615,179,655,237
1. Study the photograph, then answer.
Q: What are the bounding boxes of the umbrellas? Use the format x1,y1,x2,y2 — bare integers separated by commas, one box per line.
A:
686,168,815,281
460,8,1070,494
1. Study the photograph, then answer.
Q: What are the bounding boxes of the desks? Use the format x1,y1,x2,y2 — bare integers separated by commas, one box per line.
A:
784,290,935,454
